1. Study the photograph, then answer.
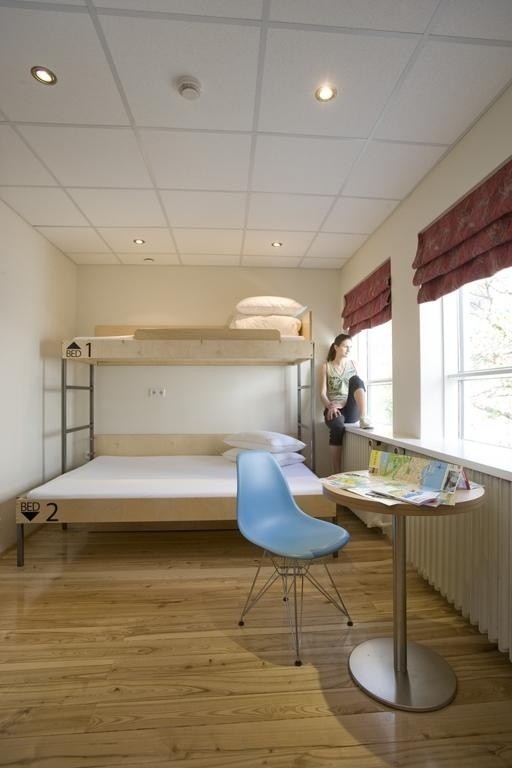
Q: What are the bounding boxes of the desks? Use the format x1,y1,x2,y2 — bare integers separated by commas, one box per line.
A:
317,466,479,713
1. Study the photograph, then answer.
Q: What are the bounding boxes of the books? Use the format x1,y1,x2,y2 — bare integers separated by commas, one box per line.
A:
371,484,441,507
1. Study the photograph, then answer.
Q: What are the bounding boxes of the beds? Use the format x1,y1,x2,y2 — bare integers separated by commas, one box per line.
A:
14,312,343,569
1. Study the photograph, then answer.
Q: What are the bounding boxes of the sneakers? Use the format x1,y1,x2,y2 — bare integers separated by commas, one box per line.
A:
360,417,374,430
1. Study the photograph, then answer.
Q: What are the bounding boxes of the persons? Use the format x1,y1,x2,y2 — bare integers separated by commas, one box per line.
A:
318,333,374,474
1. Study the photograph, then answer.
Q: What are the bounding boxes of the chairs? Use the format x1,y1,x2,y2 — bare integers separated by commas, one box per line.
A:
230,450,351,666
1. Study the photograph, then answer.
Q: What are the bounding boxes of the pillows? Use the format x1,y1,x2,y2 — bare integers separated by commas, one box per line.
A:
220,445,304,467
232,316,301,337
233,295,309,315
221,429,309,451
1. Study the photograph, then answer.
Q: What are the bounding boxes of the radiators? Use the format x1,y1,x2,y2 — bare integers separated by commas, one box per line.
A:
392,445,512,655
342,434,391,531
